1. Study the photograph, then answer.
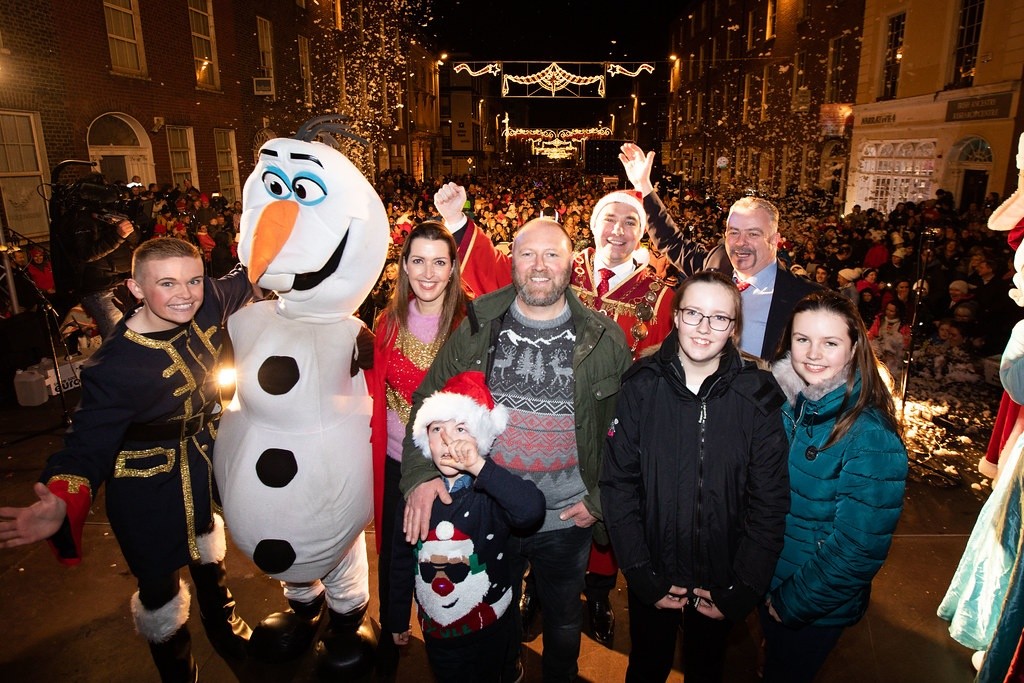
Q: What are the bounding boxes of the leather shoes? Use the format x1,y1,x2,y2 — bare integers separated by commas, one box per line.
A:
587,600,614,643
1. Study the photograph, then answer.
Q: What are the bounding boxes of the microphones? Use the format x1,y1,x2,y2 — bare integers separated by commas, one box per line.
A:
79,183,118,203
7,251,16,261
922,227,942,238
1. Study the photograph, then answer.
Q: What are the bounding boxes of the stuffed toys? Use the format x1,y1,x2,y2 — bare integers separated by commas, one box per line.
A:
111,115,390,683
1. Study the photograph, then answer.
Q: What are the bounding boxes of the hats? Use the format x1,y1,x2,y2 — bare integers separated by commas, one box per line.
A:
950,280,968,294
892,248,906,260
892,232,904,245
412,370,508,461
862,267,873,279
913,279,929,295
590,190,646,240
839,268,861,281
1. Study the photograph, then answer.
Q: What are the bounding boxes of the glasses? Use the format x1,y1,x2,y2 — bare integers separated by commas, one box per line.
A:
899,285,909,289
677,308,737,332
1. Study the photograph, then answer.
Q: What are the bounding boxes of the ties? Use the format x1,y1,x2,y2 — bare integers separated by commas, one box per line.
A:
735,279,751,291
594,268,616,311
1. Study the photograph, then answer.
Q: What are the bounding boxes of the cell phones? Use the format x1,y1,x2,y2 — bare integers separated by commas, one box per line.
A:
212,193,219,196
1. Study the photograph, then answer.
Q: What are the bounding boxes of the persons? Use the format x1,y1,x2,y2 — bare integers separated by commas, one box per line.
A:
363,143,909,683
0,237,256,683
0,165,1016,360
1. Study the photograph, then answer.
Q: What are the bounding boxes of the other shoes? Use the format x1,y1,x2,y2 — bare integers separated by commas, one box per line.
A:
519,593,542,643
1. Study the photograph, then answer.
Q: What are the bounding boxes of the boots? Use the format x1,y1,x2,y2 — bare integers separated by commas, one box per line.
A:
186,513,265,683
131,580,198,683
500,662,524,683
